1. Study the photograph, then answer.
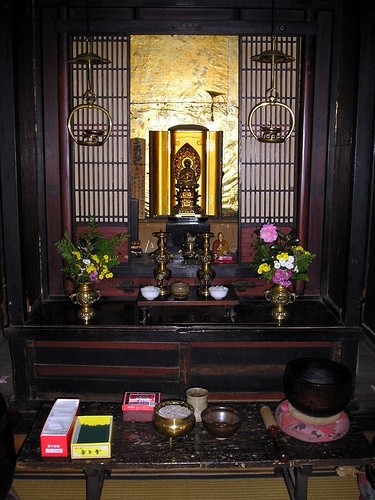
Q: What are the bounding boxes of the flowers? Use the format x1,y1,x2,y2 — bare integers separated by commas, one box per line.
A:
251,217,315,288
55,217,131,282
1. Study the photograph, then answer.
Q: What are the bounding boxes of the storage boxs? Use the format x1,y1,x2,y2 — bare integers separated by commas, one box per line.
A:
121,391,162,422
40,399,81,458
71,415,113,459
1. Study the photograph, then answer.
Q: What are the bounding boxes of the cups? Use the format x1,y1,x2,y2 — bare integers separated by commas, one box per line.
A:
186,388,208,423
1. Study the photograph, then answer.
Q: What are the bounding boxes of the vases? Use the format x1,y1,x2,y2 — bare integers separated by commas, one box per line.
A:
69,282,101,306
264,285,296,308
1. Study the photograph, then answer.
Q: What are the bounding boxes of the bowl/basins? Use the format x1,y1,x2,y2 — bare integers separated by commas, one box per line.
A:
283,358,352,417
169,282,191,298
208,285,229,299
140,286,161,300
200,406,242,440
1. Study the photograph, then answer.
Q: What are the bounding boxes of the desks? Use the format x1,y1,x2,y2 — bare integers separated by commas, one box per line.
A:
13,403,375,500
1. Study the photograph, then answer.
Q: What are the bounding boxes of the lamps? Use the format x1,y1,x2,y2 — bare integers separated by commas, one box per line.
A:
65,0,113,146
248,0,295,143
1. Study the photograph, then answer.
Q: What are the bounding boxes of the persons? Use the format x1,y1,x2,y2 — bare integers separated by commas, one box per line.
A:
177,160,196,185
212,232,230,257
